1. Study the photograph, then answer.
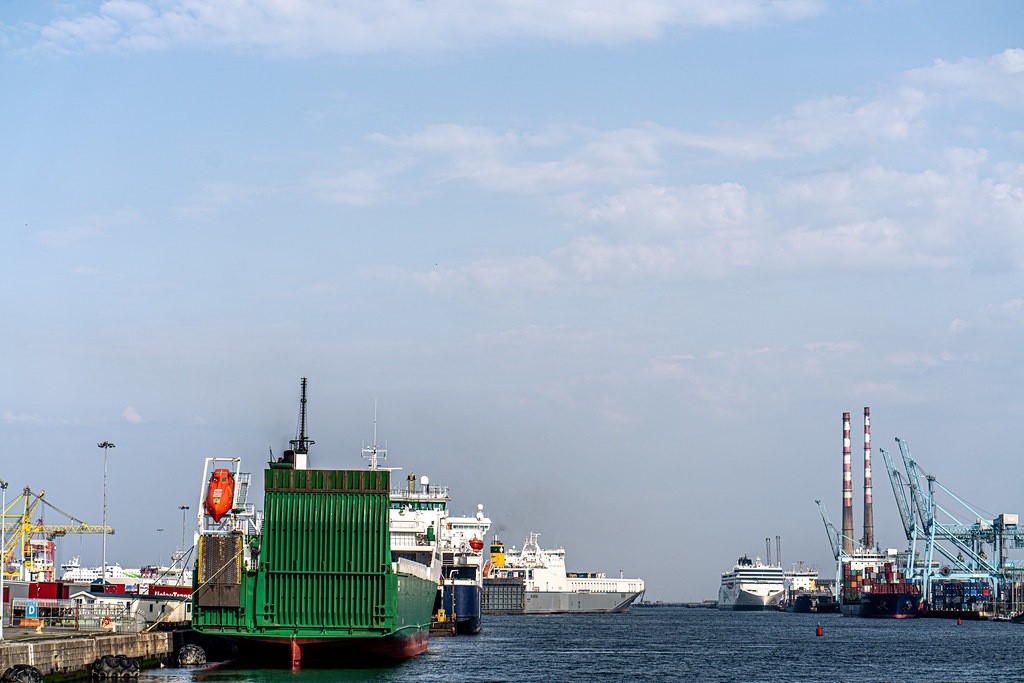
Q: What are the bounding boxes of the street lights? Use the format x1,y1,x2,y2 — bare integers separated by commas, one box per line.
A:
0,479,8,641
97,441,116,583
156,528,164,566
179,505,190,552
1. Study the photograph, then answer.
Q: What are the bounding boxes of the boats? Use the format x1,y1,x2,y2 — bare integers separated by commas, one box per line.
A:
786,589,840,614
428,503,493,634
483,531,646,612
784,561,818,591
715,556,786,609
189,374,450,673
992,614,1012,622
836,531,922,618
59,555,192,583
